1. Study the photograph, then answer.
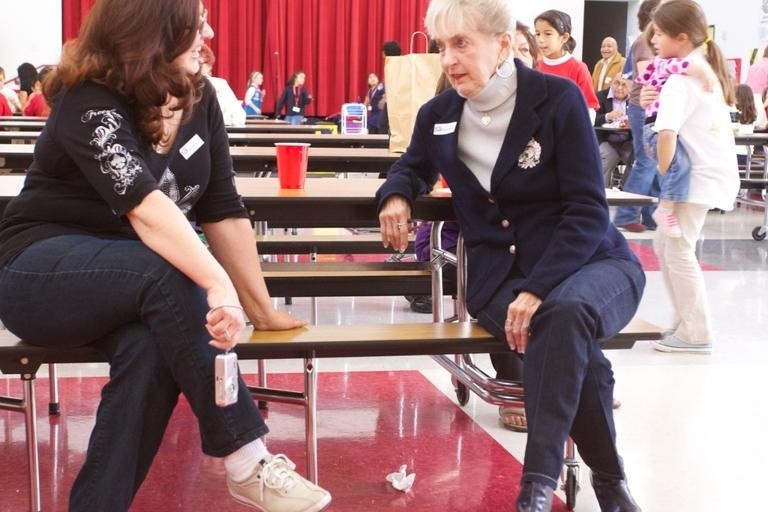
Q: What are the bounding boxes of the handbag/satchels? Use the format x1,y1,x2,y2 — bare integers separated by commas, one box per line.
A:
385,32,449,152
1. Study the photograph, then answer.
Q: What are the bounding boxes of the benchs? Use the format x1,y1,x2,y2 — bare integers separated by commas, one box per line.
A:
0,315,663,512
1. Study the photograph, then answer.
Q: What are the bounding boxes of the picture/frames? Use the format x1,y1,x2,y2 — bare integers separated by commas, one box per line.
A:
708,24,715,40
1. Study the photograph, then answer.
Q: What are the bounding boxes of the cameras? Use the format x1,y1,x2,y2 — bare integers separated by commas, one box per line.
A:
215,352,239,407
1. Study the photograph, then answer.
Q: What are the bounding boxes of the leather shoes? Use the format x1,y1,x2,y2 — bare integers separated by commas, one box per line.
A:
592,472,641,512
514,480,551,511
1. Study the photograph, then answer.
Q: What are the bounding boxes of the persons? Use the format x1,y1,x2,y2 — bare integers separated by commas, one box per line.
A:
724,78,756,200
274,71,313,125
0,0,331,512
0,44,266,127
613,2,740,354
364,0,649,511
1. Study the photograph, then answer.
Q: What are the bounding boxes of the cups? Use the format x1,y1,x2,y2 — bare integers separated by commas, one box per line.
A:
274,142,311,189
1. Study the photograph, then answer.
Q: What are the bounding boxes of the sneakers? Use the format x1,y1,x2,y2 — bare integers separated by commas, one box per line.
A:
656,327,713,353
226,456,332,512
405,292,432,313
615,209,681,238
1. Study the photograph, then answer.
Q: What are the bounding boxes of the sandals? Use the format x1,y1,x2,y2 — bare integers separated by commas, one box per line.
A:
499,405,527,432
